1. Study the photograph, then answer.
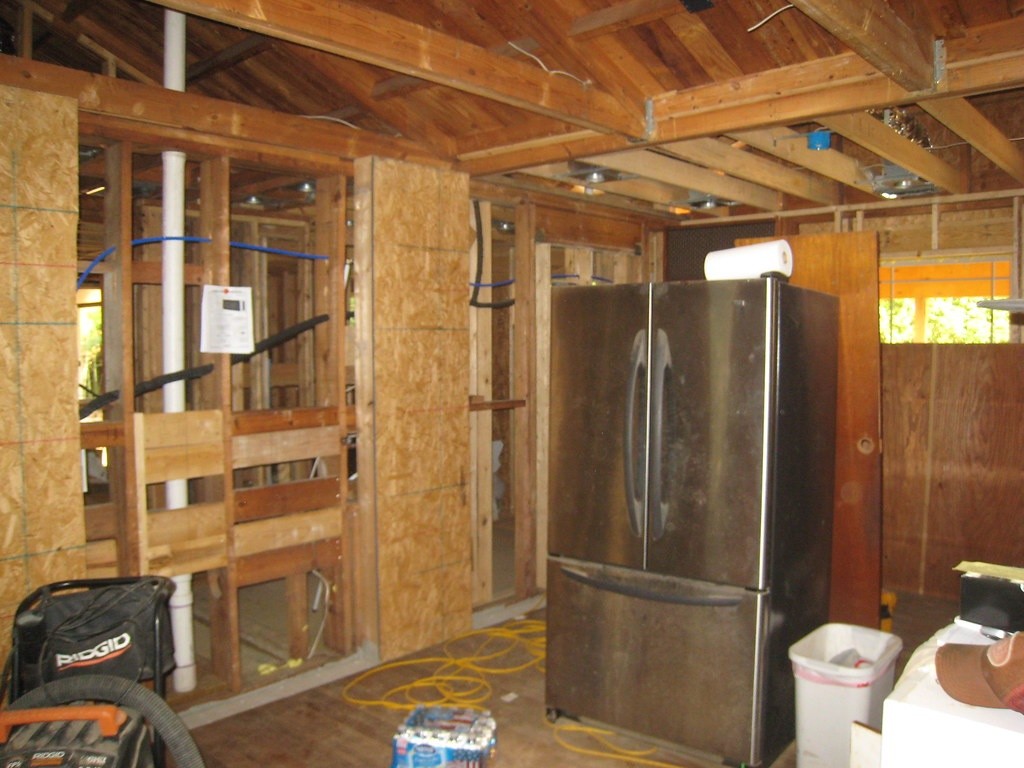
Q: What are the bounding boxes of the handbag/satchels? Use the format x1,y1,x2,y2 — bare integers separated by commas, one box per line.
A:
9,575,177,707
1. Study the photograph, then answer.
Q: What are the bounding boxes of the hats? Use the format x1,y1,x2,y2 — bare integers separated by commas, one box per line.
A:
935,631,1024,714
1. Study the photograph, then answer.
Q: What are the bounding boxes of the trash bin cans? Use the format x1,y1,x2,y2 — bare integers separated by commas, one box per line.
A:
787,621,904,768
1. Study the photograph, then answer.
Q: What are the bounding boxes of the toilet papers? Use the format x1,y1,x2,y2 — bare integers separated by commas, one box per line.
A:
703,238,792,281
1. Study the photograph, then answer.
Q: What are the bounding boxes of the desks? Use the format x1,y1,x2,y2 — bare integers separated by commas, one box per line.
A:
881,625,1024,768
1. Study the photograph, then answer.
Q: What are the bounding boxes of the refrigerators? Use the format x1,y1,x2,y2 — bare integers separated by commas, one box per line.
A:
546,273,843,768
